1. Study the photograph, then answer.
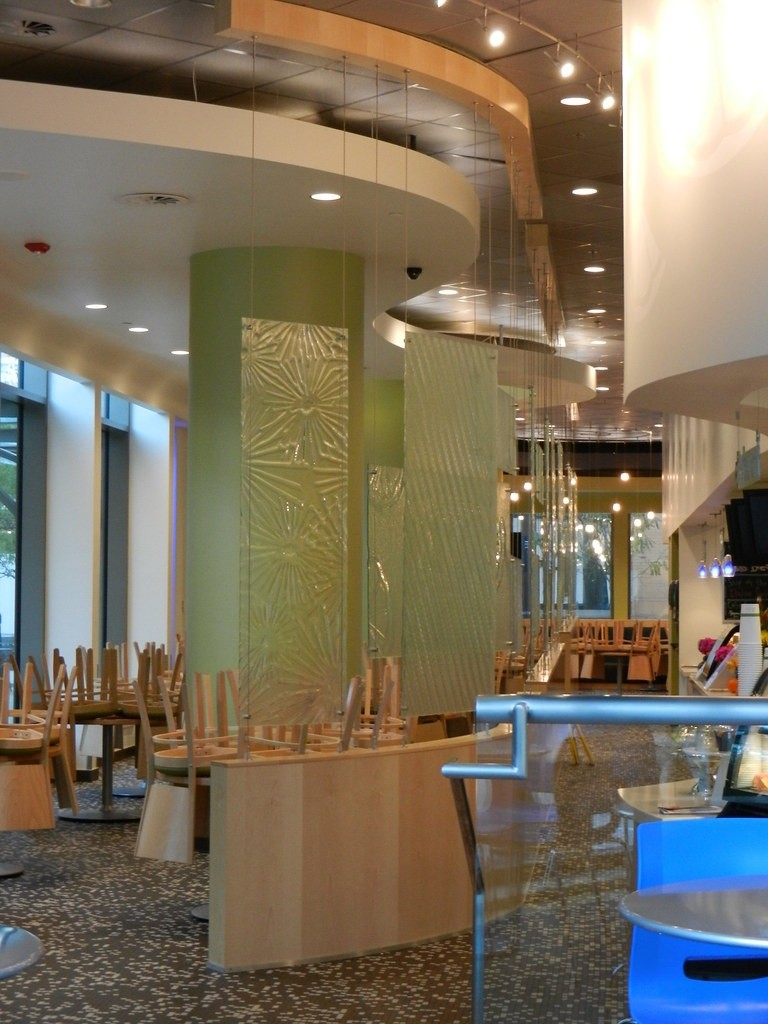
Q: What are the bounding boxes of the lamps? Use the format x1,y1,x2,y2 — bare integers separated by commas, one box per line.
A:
698,522,710,579
719,503,736,578
709,510,722,580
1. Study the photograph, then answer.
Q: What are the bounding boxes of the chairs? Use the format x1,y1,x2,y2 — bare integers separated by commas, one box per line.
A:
494,613,670,694
0,633,415,866
626,818,768,1024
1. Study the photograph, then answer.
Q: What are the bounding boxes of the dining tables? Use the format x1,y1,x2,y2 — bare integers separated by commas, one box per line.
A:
618,873,768,950
